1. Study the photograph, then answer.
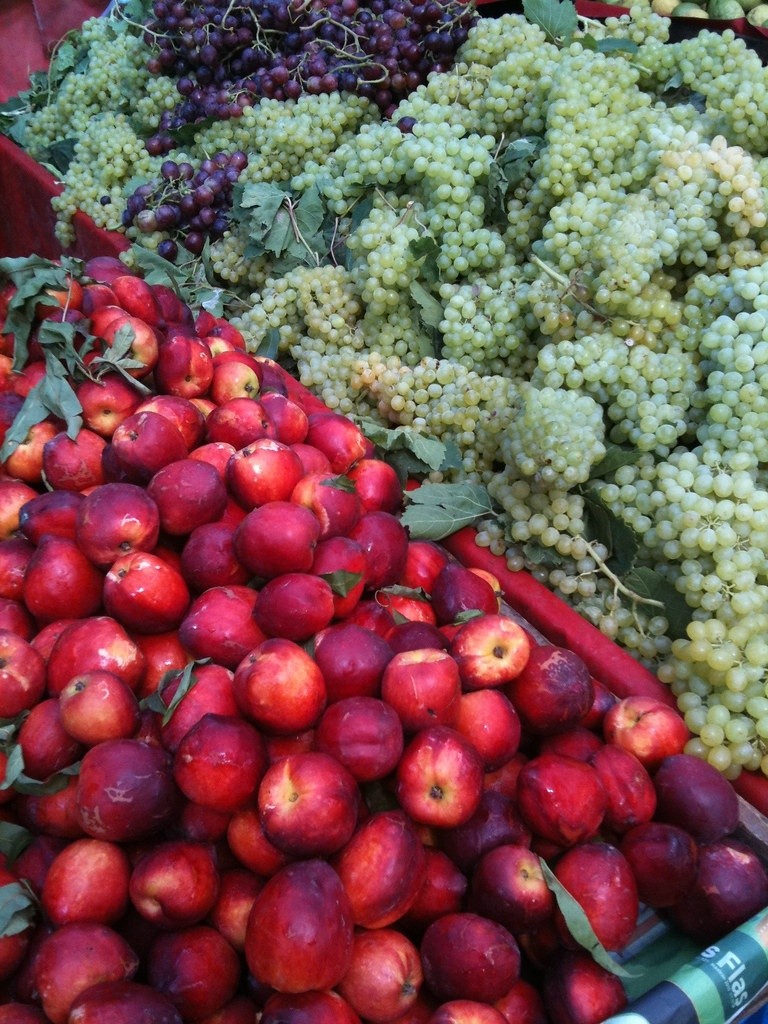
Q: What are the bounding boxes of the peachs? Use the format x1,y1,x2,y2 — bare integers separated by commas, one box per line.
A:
0,256,768,1024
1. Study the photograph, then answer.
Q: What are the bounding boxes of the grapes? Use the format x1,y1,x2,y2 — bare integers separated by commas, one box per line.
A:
20,0,768,788
